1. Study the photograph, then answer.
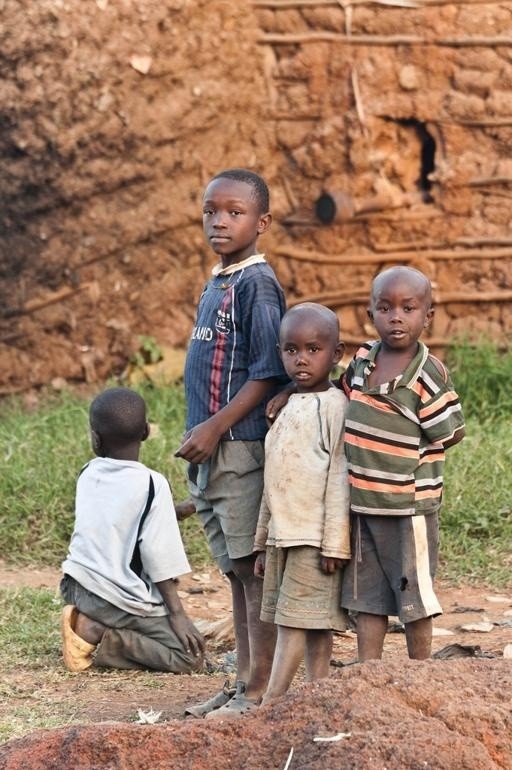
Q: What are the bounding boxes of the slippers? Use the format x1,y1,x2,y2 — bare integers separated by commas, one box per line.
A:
184,679,237,718
61,604,96,673
204,680,258,718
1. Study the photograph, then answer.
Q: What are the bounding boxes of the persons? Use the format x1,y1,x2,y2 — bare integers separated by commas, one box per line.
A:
262,265,469,665
174,170,289,724
57,386,212,677
254,301,353,710
